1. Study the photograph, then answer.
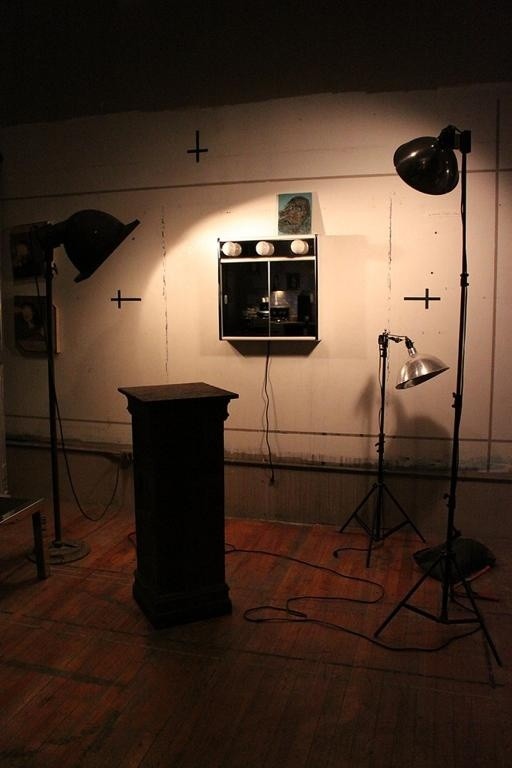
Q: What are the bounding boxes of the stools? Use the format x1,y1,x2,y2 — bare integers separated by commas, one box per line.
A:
1,494,52,580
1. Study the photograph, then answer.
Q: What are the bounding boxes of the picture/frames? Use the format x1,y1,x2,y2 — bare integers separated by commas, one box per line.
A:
9,219,51,282
12,293,65,359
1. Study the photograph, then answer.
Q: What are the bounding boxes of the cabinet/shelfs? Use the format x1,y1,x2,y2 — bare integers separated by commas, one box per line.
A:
215,233,322,343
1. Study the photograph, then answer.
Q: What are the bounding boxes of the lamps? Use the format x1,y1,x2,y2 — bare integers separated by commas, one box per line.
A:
373,123,503,667
26,208,141,566
339,329,451,568
